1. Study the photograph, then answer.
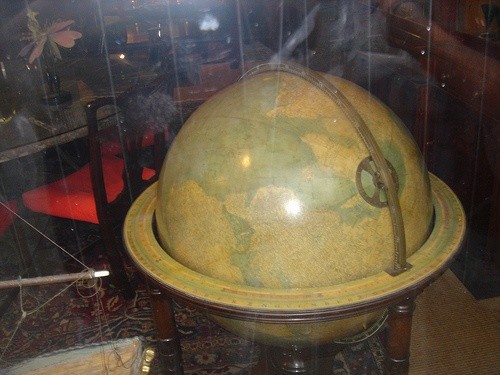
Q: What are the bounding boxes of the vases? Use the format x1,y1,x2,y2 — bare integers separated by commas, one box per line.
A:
31,73,74,135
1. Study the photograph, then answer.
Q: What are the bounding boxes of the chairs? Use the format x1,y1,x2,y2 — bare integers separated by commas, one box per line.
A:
21,81,177,302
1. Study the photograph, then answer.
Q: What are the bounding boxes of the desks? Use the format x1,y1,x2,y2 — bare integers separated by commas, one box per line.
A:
0,56,169,268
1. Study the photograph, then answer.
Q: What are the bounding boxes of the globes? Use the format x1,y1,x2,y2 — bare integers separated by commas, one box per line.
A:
122,60,465,375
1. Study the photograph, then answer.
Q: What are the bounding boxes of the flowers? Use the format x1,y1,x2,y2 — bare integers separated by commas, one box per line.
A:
18,6,83,77
